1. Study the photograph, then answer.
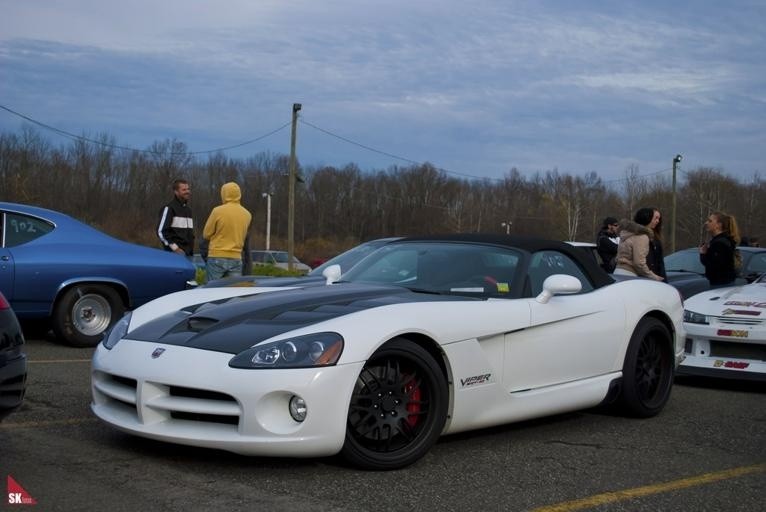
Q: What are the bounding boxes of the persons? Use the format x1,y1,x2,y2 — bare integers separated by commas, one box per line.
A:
202,181,252,282
156,178,196,263
612,207,665,281
597,217,622,274
645,206,668,283
699,211,741,289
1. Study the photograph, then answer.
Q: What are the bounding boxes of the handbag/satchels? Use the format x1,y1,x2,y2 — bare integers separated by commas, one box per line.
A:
733,248,743,278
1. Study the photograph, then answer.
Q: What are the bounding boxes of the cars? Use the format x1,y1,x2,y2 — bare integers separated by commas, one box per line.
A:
563,240,613,273
676,273,766,386
193,254,206,271
0,200,198,349
241,248,312,275
658,243,764,300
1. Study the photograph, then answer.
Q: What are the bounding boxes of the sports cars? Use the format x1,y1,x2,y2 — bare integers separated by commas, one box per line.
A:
89,233,687,469
